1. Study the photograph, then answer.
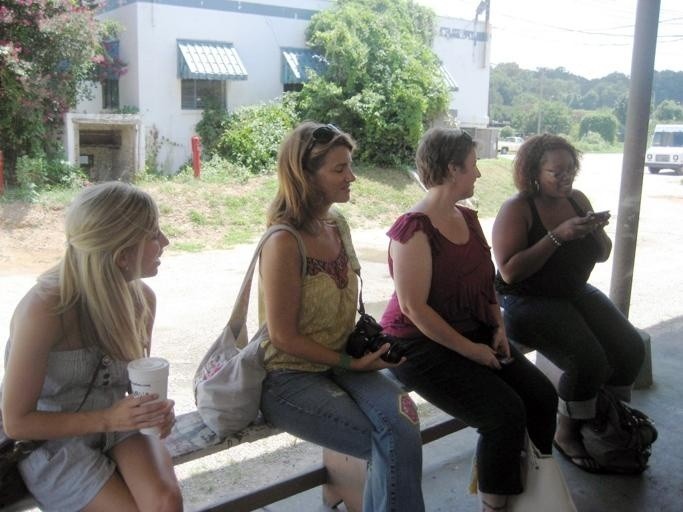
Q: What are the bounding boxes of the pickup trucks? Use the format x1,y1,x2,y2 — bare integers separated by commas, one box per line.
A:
494,136,524,154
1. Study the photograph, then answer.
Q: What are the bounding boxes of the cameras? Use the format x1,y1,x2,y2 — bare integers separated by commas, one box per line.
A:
346,315,401,362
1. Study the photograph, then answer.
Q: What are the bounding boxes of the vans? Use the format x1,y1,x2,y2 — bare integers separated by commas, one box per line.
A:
644,124,683,175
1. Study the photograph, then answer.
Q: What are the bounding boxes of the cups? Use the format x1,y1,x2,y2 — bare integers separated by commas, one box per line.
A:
127,358,170,436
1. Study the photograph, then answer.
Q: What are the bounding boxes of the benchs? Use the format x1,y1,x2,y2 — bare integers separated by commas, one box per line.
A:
0,338,564,512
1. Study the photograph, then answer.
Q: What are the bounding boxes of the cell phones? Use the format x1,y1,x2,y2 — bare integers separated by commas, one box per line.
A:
589,210,610,227
493,352,513,366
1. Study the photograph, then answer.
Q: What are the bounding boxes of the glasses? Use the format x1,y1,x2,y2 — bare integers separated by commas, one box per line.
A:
304,123,341,166
539,164,579,179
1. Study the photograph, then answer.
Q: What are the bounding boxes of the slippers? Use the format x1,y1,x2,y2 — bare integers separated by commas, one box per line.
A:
552,438,603,475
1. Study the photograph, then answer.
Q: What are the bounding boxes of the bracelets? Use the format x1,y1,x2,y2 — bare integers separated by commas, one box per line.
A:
547,230,563,247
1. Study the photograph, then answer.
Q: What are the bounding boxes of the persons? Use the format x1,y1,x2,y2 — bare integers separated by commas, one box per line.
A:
244,121,425,512
378,127,560,512
0,177,186,512
491,131,656,476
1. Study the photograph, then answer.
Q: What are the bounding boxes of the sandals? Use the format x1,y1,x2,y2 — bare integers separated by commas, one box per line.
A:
482,495,508,511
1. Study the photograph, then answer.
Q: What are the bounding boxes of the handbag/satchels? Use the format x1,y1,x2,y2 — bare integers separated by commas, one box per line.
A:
194,321,267,439
580,387,658,476
0,440,48,508
